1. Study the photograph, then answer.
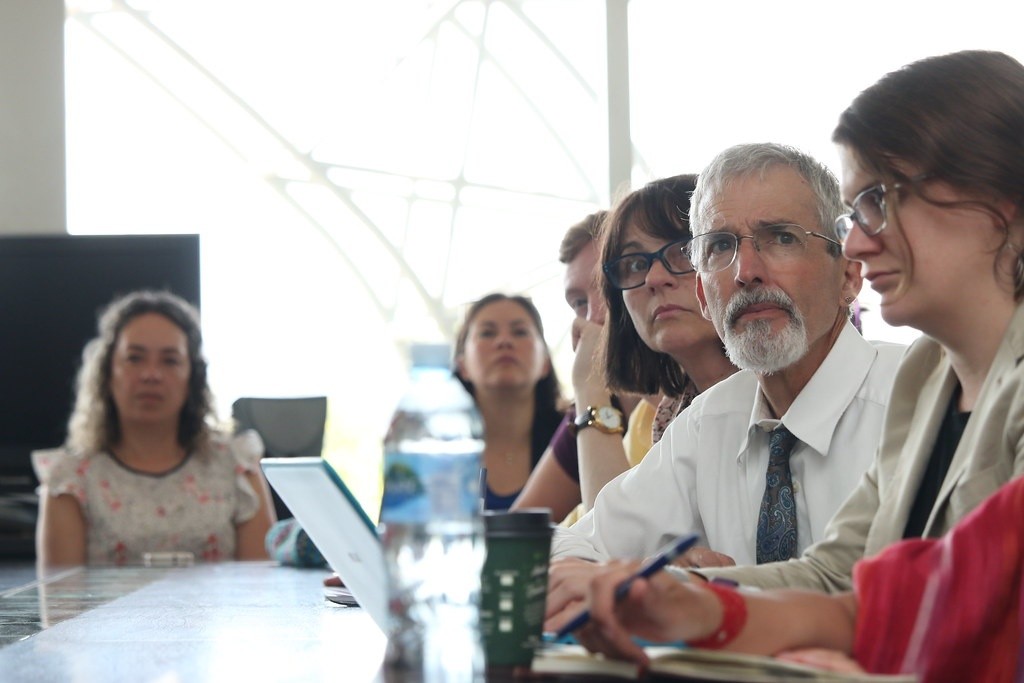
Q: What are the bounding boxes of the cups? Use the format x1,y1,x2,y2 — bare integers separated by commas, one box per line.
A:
479,508,553,683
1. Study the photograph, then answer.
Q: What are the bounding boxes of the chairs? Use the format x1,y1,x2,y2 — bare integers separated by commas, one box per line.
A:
231,395,329,520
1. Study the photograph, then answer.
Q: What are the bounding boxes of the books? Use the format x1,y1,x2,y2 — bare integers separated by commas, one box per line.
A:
531,640,913,683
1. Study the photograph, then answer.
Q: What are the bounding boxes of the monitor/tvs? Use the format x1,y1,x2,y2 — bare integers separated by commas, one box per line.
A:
0,236,201,487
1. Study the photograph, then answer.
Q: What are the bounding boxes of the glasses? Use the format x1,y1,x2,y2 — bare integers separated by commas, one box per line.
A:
834,171,937,245
603,237,695,290
681,224,840,273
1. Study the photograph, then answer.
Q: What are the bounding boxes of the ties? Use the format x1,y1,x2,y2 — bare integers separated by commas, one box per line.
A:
756,427,798,565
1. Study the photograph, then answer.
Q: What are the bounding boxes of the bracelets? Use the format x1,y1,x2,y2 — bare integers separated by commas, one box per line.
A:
688,578,748,648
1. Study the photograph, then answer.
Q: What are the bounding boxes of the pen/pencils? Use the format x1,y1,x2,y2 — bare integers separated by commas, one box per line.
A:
552,534,699,643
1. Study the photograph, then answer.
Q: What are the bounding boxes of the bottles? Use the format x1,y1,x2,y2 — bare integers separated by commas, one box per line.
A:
380,342,488,683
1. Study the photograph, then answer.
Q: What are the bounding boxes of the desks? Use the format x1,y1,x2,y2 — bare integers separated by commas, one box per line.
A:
0,560,708,681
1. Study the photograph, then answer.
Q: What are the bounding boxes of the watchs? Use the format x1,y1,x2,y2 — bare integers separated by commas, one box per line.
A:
572,406,625,433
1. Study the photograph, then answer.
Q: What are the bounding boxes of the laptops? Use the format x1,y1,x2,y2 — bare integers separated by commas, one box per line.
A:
257,458,425,662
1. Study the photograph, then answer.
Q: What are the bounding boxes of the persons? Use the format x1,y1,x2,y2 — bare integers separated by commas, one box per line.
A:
570,476,1024,683
452,291,569,512
545,51,1024,629
33,293,277,563
601,174,744,445
552,144,910,572
510,209,669,528
